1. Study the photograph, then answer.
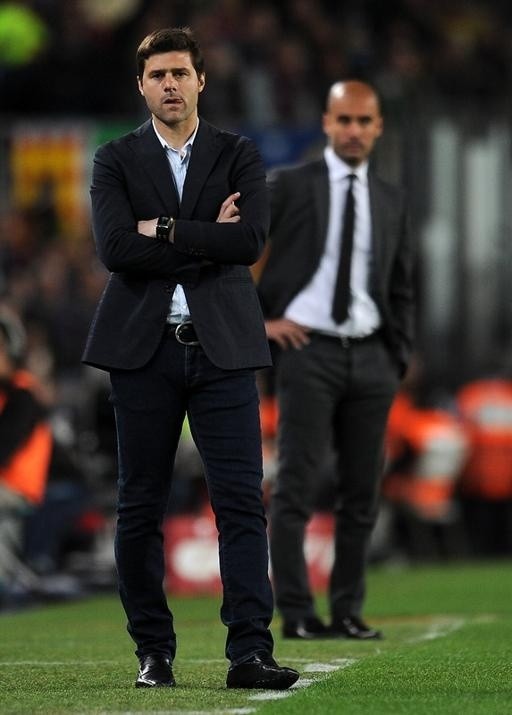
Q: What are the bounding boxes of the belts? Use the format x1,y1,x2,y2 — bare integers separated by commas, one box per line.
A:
164,321,200,346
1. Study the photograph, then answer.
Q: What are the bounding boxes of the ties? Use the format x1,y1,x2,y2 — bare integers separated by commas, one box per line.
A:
333,174,356,323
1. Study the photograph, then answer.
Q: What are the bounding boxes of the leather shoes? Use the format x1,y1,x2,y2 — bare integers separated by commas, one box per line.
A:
330,614,379,639
135,651,176,687
227,649,299,690
284,616,330,639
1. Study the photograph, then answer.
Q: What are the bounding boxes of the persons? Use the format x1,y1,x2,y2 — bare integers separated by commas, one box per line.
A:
81,22,300,691
1,0,512,612
255,80,419,641
